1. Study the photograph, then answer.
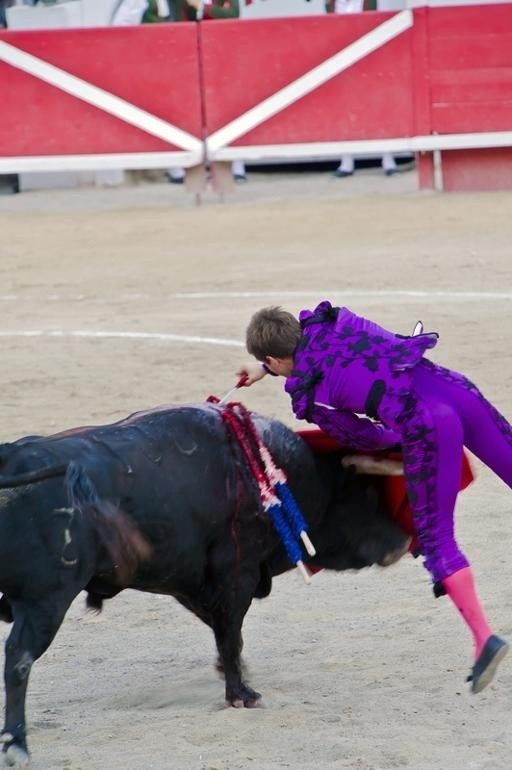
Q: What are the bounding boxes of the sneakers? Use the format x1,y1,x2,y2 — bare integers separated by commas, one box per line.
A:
164,171,183,183
471,634,508,694
232,173,246,182
328,169,353,177
384,162,415,175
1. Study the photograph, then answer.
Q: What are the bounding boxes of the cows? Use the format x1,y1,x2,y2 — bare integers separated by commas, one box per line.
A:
1,397,418,770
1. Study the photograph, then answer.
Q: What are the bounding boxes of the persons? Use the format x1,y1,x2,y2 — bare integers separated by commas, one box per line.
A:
233,297,512,694
177,0,251,183
110,0,188,183
323,1,401,180
